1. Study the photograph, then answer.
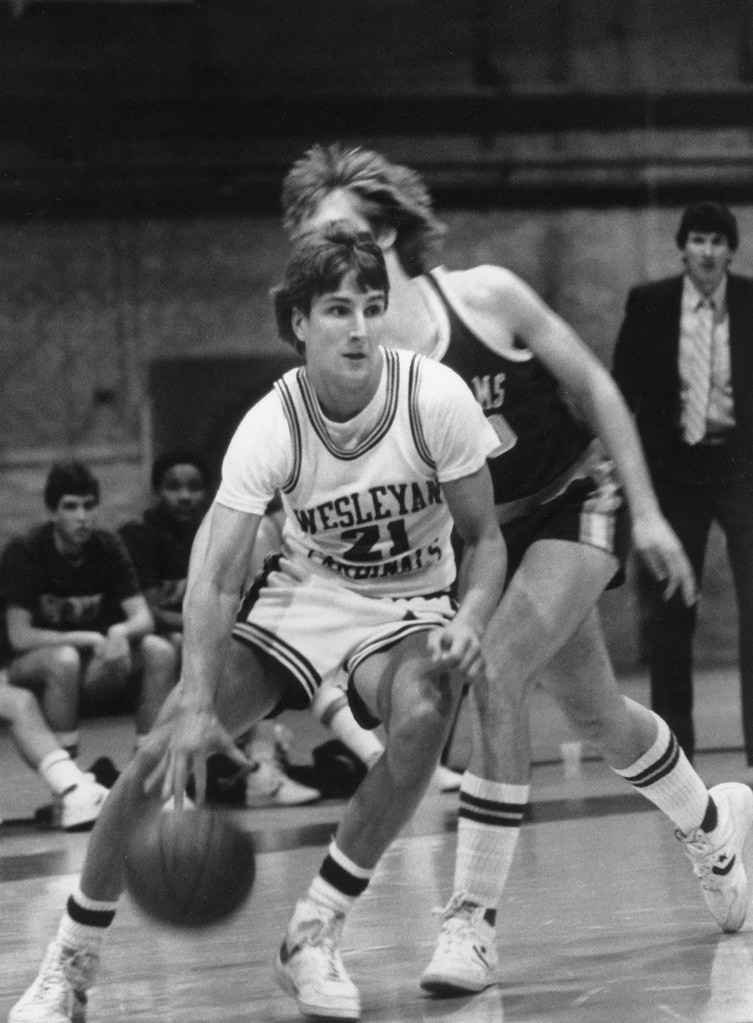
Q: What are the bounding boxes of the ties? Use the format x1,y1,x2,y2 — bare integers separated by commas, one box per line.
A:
684,298,714,445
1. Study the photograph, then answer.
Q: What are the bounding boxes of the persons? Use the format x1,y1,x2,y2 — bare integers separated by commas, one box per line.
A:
606,202,752,770
0,140,753,1023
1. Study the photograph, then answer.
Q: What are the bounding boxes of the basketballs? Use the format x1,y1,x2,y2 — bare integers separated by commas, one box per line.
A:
123,807,257,930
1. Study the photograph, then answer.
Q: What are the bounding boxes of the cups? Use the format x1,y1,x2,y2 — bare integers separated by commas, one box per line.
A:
561,743,581,778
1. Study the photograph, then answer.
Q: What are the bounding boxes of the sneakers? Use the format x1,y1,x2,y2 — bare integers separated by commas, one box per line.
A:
673,781,753,932
62,775,113,825
7,938,105,1023
429,764,464,790
275,898,361,1019
419,892,500,992
242,764,320,808
